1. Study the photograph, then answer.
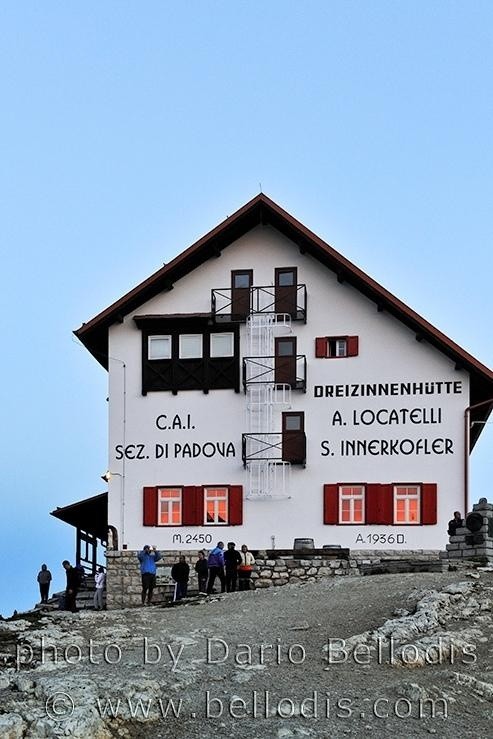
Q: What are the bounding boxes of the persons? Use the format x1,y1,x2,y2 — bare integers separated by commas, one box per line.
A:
61,560,81,612
172,542,256,599
138,544,162,606
93,567,106,611
37,564,52,604
